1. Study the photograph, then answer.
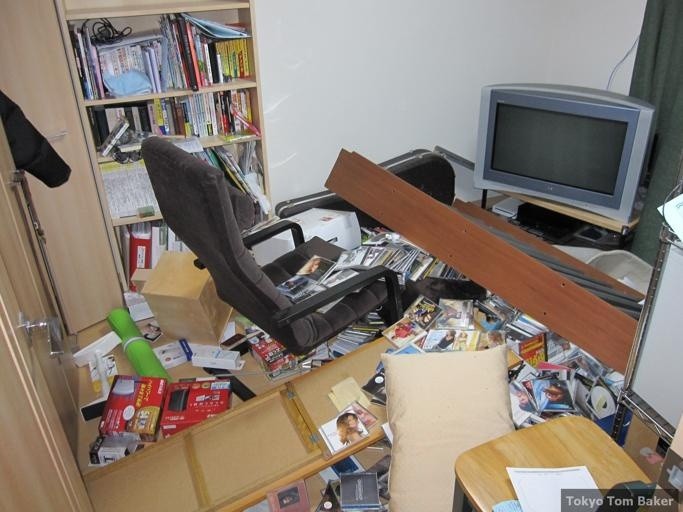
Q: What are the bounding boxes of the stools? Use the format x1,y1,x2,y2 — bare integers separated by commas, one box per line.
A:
451,415,681,510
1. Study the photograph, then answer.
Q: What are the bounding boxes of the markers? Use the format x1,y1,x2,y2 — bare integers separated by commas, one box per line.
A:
179,376,218,383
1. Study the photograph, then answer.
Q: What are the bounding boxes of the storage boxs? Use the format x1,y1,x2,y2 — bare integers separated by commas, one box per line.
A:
622,412,682,486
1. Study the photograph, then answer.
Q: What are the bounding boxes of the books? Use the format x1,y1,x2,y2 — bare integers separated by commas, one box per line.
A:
66,12,272,294
243,224,616,458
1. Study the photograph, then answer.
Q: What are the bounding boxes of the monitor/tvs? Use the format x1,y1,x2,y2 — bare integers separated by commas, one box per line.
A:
471,82,656,224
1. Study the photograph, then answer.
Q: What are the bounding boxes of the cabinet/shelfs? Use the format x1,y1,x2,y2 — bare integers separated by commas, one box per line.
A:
611,177,683,447
480,188,640,250
0,0,271,512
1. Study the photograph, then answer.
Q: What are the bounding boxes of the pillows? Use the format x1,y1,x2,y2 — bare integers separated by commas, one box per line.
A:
382,342,516,512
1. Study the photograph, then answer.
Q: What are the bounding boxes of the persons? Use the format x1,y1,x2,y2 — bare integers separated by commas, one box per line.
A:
279,489,301,508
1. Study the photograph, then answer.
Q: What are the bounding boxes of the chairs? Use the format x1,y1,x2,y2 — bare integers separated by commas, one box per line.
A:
141,135,405,402
